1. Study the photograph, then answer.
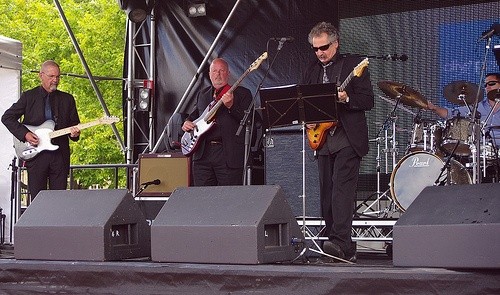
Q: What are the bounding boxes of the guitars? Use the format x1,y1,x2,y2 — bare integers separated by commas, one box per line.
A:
13,117,120,160
305,57,369,151
180,51,268,156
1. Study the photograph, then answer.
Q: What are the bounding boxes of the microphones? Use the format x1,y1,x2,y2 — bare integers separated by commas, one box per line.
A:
142,179,161,185
269,36,295,43
386,54,408,62
481,23,500,40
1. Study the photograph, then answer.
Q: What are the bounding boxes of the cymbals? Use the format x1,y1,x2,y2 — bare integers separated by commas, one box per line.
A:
488,126,500,129
443,81,484,106
377,80,428,109
487,88,500,102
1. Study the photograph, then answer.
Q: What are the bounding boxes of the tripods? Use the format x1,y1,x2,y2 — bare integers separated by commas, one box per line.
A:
355,93,403,217
260,82,356,265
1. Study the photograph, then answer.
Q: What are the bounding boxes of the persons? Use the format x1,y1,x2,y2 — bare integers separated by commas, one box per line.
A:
296,22,374,261
2,60,80,203
428,73,500,184
181,58,256,188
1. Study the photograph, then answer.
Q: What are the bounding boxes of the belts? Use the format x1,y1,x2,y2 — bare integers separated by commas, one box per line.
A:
210,140,222,144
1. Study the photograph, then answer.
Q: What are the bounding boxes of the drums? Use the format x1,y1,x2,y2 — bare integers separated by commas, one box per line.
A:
476,141,498,160
412,119,441,148
444,116,479,156
390,151,474,215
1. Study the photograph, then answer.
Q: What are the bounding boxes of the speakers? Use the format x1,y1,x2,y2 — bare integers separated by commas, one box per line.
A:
392,183,500,269
14,132,322,265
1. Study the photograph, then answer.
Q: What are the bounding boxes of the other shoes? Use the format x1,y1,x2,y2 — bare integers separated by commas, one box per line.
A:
322,241,344,263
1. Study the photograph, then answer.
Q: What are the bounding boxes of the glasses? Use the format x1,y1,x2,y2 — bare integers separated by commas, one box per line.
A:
311,42,333,52
483,80,500,88
